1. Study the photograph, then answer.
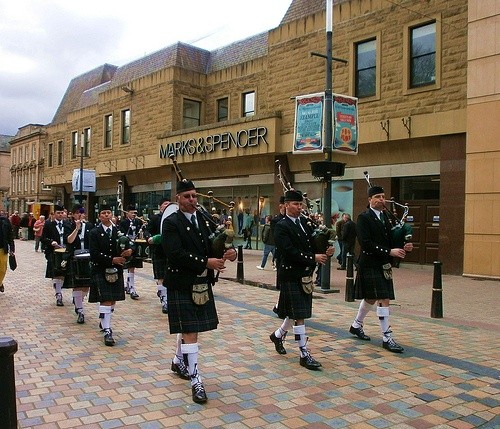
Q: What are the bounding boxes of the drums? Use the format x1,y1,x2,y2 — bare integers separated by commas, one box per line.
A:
131,239,149,259
72,253,92,280
54,248,67,270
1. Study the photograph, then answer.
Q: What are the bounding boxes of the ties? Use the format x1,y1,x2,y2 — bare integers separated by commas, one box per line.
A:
106,228,111,235
191,215,196,226
295,219,306,238
58,222,62,230
380,212,384,222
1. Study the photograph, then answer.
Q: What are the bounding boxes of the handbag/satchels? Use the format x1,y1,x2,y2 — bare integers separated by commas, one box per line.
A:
33,226,41,232
302,276,314,294
9,253,17,271
192,283,209,305
383,263,392,279
105,268,118,282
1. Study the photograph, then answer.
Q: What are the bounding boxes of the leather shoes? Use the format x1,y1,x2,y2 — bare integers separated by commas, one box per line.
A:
35,246,357,402
349,325,370,340
383,338,403,352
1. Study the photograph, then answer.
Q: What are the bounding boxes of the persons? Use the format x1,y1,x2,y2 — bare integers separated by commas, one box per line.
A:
335,213,359,271
349,186,413,351
273,195,286,318
244,209,254,249
0,209,36,240
0,216,15,292
258,212,339,284
34,216,46,252
161,179,237,403
44,205,89,325
87,205,134,348
114,202,172,313
270,190,335,368
211,206,228,227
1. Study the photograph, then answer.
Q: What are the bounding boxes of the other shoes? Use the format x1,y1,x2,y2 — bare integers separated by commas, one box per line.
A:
256,266,263,269
0,284,4,292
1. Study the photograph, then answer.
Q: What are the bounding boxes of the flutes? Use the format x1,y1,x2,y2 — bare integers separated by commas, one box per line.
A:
364,170,414,267
108,180,150,265
274,159,337,254
167,151,235,287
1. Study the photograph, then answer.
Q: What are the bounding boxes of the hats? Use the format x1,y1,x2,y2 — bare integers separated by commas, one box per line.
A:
128,206,137,211
369,186,384,197
54,205,64,212
98,205,112,214
279,196,286,204
158,198,170,206
284,191,303,202
177,181,195,193
